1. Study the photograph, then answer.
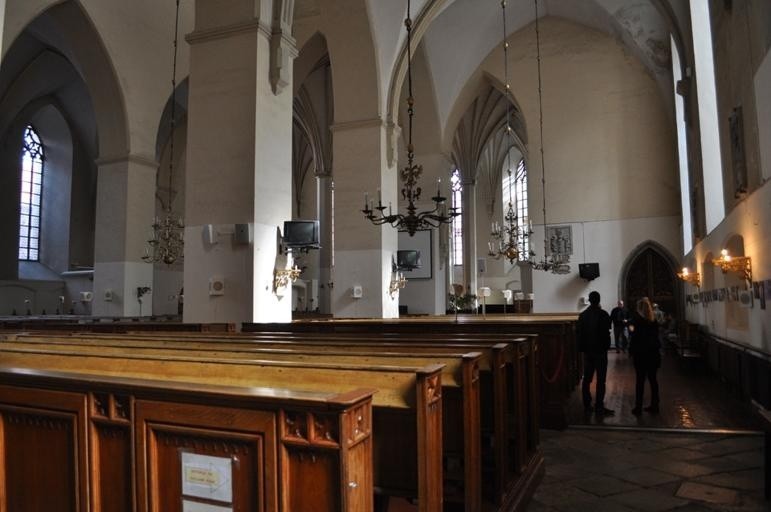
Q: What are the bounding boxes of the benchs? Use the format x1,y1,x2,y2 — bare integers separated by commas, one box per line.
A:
1,314,583,509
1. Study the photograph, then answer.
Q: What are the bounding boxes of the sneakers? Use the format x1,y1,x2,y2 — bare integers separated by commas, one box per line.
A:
632,404,659,415
585,405,614,415
616,348,627,353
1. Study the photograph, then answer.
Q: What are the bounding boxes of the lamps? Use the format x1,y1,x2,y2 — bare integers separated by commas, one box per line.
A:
713,251,752,286
678,267,702,287
361,0,464,234
141,0,186,266
489,1,570,275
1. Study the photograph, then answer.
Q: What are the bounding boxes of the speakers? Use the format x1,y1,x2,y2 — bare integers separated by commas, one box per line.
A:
233,223,253,245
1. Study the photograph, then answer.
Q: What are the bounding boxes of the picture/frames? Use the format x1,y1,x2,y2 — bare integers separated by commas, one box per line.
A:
726,105,750,198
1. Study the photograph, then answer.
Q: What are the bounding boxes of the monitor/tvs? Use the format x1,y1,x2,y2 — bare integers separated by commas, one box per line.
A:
284,220,319,246
397,249,422,268
578,262,601,281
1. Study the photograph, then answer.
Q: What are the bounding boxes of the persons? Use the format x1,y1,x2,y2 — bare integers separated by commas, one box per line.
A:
651,302,664,327
609,300,629,354
659,313,676,348
621,297,661,417
576,291,615,416
